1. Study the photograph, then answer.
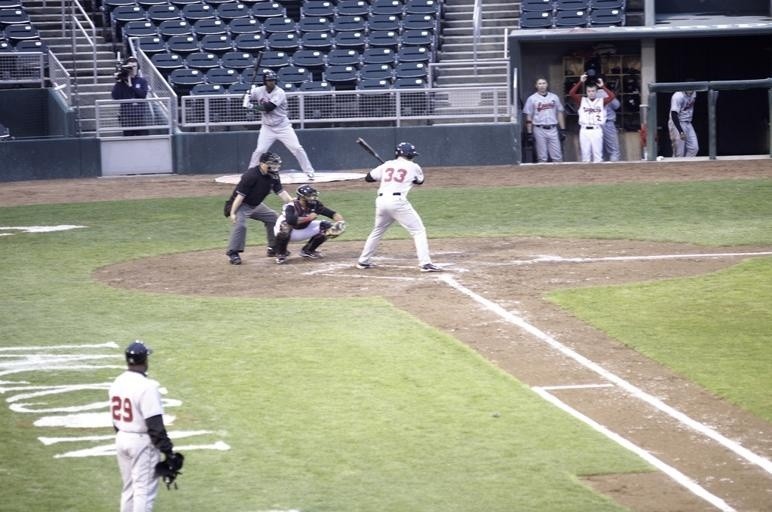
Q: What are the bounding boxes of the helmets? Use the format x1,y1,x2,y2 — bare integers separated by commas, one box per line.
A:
124,341,154,365
263,68,278,81
395,141,421,159
260,151,282,176
296,184,321,206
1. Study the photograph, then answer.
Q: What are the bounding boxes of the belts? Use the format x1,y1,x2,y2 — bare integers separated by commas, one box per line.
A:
376,192,402,197
584,126,595,130
536,124,558,130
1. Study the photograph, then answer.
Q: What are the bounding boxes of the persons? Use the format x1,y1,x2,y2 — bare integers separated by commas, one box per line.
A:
668,91,703,157
107,342,177,512
243,72,316,180
355,142,443,272
522,75,566,162
273,185,346,264
224,152,297,265
569,72,616,161
594,74,622,161
112,58,148,136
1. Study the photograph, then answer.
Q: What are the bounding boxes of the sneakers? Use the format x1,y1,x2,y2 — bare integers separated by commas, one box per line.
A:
298,248,326,260
276,251,292,264
229,253,243,266
420,263,444,273
356,261,378,270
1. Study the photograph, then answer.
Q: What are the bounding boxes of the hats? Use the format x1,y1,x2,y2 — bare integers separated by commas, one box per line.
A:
586,68,596,77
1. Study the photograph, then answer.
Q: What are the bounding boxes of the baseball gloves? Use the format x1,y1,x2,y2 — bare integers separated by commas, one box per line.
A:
152,453,183,490
326,221,346,239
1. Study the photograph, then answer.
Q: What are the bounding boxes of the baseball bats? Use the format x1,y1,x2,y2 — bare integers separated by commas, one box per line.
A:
249,51,264,92
356,137,385,164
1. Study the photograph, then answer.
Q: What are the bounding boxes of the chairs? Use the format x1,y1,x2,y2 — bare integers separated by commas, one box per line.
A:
519,0,625,29
0,0,50,87
